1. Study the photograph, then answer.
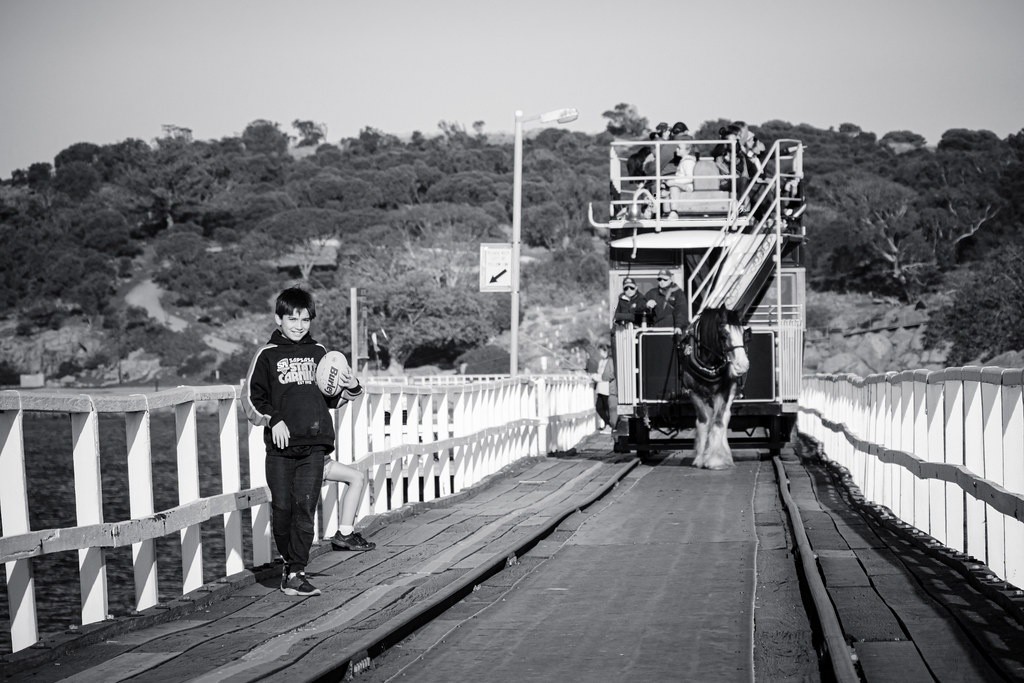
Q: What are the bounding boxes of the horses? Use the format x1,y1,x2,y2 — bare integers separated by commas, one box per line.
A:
676,303,749,469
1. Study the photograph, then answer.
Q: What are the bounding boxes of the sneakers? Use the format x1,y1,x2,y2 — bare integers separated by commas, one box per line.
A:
285,570,321,596
331,530,376,551
281,573,287,592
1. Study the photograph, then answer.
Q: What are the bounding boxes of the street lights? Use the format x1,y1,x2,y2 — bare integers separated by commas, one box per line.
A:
510,108,580,380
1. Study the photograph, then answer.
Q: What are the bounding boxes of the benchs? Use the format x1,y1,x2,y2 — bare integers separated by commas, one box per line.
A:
663,157,750,212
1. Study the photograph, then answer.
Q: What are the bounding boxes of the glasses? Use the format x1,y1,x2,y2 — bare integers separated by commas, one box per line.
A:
657,278,667,281
624,287,635,291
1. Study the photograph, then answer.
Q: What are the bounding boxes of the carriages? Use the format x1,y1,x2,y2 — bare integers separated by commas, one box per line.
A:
589,132,806,462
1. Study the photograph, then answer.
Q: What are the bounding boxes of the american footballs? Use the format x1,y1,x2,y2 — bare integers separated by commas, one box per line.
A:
315,350,349,398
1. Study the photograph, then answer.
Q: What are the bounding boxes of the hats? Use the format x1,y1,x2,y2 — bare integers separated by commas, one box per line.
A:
623,277,636,286
658,269,674,275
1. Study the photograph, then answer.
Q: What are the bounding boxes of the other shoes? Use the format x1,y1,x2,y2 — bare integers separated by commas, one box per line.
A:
600,425,612,435
598,419,605,430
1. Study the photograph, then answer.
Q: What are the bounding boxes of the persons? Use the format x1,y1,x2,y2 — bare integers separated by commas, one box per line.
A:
610,278,645,335
323,455,376,551
626,121,808,221
643,270,690,335
240,287,363,595
588,343,617,435
